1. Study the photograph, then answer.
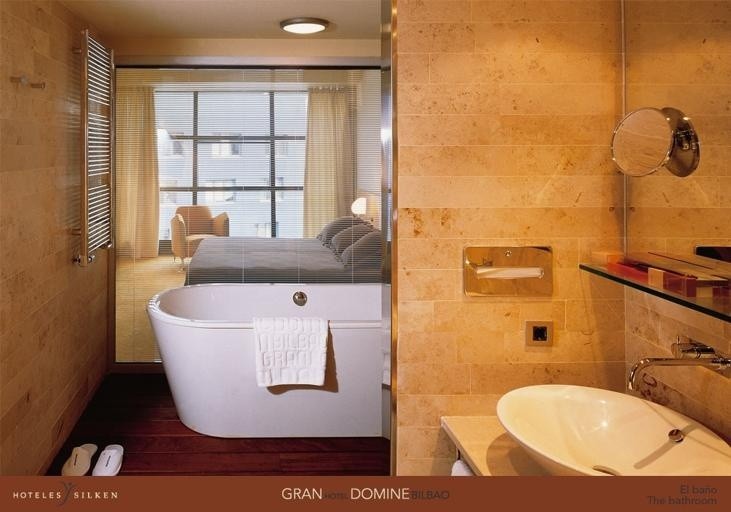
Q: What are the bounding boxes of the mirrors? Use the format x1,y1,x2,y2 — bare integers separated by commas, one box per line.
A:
611,106,675,176
662,108,700,177
622,0,731,288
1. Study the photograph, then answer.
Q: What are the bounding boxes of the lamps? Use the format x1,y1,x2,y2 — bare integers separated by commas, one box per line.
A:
280,18,327,34
351,198,366,214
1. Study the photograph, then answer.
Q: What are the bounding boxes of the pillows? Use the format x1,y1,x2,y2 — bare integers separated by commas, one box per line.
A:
316,217,381,268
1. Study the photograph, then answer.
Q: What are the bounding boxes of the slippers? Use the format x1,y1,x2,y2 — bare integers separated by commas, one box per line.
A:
91,444,125,477
61,443,98,477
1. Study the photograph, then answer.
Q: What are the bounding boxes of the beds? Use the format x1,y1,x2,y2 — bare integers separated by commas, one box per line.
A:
184,191,382,286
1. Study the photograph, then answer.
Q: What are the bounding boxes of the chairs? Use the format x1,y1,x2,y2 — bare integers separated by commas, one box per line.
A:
171,206,229,264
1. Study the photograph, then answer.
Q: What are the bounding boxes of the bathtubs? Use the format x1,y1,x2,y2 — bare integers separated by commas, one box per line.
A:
146,283,385,438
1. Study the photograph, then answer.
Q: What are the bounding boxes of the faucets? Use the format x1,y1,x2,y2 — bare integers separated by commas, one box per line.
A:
627,356,719,389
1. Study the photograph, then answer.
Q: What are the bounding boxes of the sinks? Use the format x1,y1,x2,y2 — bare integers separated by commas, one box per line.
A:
495,384,731,479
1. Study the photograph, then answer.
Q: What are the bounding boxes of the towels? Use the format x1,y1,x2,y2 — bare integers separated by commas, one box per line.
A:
451,460,473,477
254,318,329,387
